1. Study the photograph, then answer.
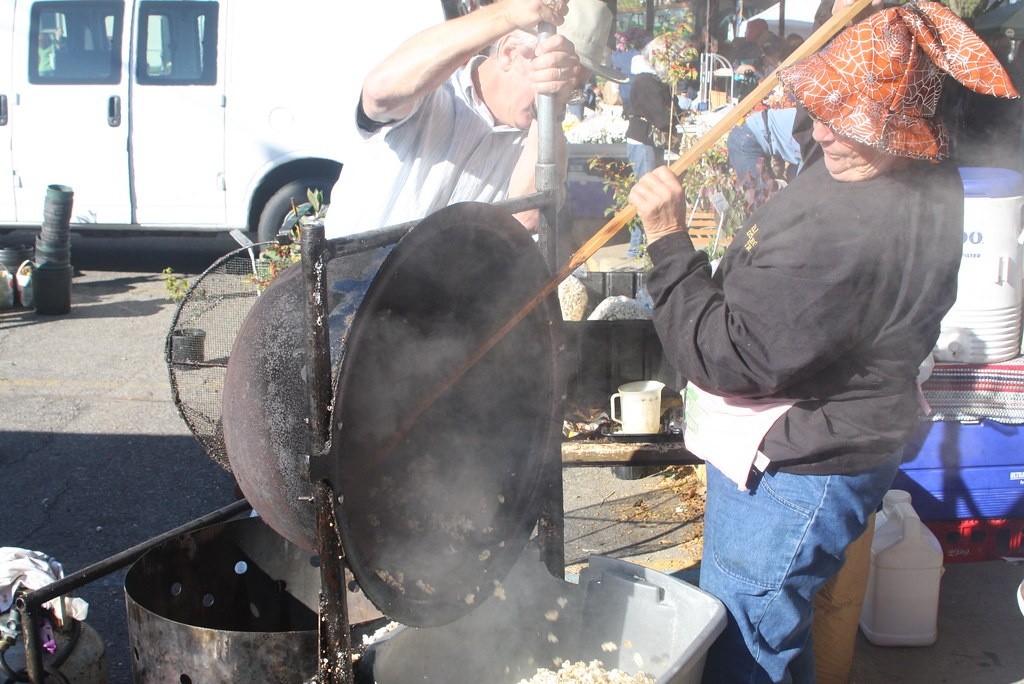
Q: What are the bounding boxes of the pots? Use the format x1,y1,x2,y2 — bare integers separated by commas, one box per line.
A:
222,202,569,630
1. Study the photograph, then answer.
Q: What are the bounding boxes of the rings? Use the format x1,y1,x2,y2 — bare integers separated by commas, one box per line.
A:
558,67,562,80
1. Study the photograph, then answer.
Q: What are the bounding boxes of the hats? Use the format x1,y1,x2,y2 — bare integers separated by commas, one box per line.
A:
520,0,631,84
776,0,1023,163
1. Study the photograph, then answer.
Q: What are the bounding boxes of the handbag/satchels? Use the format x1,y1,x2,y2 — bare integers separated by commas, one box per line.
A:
649,123,681,150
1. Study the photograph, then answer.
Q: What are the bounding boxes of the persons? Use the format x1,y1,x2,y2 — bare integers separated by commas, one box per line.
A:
726,104,801,199
627,0,965,684
933,17,1024,153
325,2,630,238
582,16,806,114
622,40,683,258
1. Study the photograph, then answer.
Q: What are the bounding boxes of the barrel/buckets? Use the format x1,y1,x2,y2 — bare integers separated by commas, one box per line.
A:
35,184,74,316
171,329,206,371
934,165,1024,363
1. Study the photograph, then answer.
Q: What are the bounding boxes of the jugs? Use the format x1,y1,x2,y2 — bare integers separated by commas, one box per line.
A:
611,380,666,434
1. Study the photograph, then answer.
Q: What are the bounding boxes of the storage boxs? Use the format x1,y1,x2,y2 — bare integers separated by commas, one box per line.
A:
926,518,1024,564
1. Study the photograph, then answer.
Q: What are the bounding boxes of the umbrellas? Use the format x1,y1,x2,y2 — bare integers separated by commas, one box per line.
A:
973,0,1024,40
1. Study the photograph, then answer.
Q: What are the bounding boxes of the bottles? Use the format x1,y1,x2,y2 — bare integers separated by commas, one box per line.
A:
860,489,944,646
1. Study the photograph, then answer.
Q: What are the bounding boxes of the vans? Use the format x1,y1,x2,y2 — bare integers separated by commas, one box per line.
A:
0,0,494,273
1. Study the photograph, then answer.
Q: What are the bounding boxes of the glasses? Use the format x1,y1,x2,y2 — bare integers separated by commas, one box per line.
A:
568,89,588,105
